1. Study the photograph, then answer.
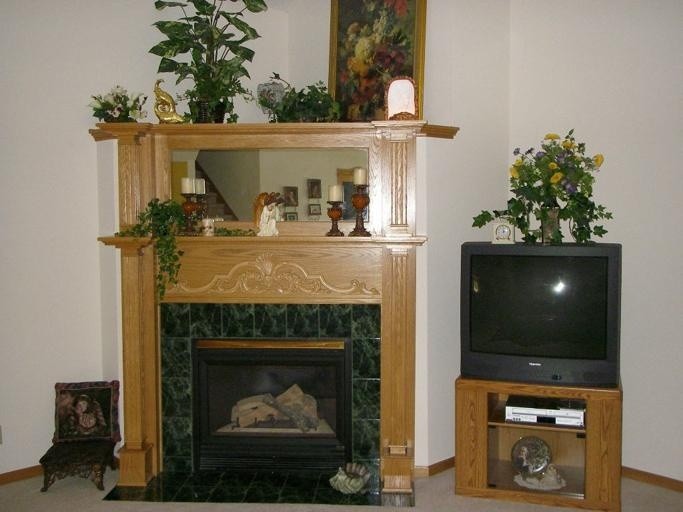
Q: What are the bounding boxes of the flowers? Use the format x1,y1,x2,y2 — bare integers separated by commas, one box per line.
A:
90,84,149,121
472,129,615,246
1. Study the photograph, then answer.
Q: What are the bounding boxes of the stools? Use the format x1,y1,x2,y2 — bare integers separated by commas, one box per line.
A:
39,443,118,493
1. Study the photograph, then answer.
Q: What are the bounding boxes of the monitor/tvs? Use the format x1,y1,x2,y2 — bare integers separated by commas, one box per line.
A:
461,243,622,389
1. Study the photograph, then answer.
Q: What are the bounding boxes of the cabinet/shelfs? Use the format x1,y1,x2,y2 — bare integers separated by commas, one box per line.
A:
453,377,623,508
86,118,427,507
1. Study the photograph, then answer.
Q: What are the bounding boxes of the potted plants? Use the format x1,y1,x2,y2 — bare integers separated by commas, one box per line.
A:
119,197,258,298
147,0,268,123
257,72,343,123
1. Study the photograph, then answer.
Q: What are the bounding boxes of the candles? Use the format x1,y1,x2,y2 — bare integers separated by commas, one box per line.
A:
353,164,369,185
328,183,344,201
193,178,207,194
180,175,195,194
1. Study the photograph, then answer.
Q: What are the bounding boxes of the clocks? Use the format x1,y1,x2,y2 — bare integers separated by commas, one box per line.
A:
490,217,516,244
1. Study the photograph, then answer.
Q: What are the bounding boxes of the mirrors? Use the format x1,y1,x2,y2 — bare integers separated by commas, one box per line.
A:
383,74,420,121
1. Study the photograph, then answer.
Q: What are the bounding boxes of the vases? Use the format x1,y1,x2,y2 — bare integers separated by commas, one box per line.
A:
110,117,134,125
537,203,564,246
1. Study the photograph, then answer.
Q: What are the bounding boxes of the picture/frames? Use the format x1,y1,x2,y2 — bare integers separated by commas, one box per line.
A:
309,203,321,215
52,380,122,444
307,177,322,198
284,185,298,207
284,212,298,221
328,0,427,122
